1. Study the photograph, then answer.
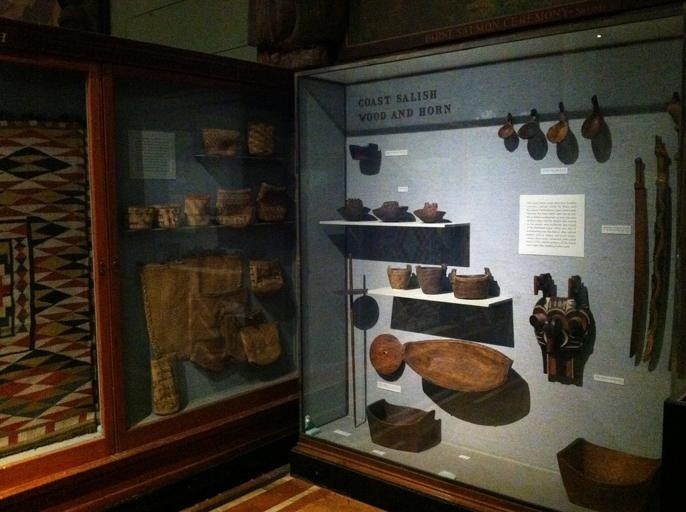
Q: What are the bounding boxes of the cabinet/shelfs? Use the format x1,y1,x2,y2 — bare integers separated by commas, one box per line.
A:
1,31,297,508
297,32,686,510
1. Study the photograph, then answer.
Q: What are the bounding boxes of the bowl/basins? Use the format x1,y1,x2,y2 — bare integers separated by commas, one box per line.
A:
337,207,370,221
414,208,447,223
372,207,408,222
